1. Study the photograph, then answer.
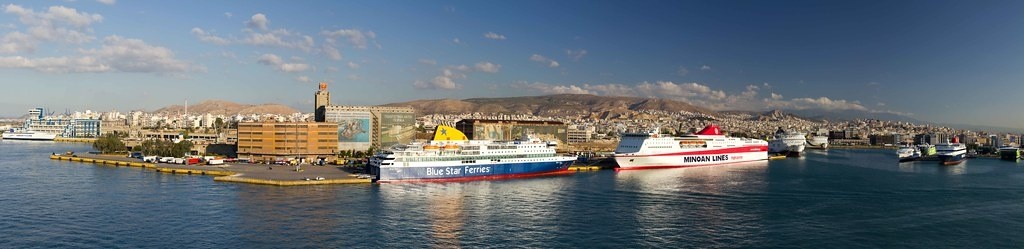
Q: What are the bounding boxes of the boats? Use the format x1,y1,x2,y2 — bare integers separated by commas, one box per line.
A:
765,126,807,154
921,144,936,156
803,134,830,149
3,129,56,141
934,134,968,165
998,146,1021,160
896,145,923,160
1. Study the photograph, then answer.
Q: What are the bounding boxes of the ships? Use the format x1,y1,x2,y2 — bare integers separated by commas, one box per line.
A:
609,122,769,168
373,123,579,185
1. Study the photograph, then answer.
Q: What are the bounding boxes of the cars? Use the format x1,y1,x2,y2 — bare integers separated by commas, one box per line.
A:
132,150,326,180
